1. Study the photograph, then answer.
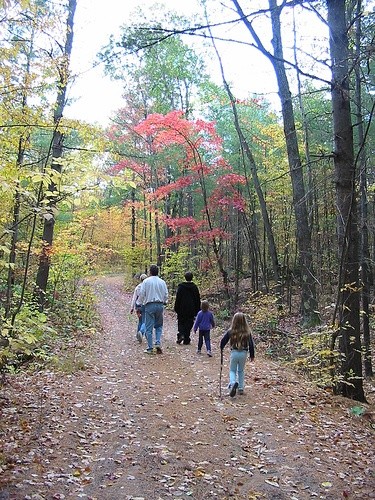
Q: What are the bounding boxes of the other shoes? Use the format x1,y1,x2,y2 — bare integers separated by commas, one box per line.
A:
184,341,189,344
176,336,184,343
144,349,153,352
156,346,162,354
138,331,143,342
196,350,201,353
207,352,212,357
238,390,243,395
230,382,239,398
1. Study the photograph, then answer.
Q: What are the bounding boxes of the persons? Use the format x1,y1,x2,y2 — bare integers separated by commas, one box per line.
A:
135,265,168,353
174,272,201,345
130,274,147,343
220,312,254,398
193,300,215,357
